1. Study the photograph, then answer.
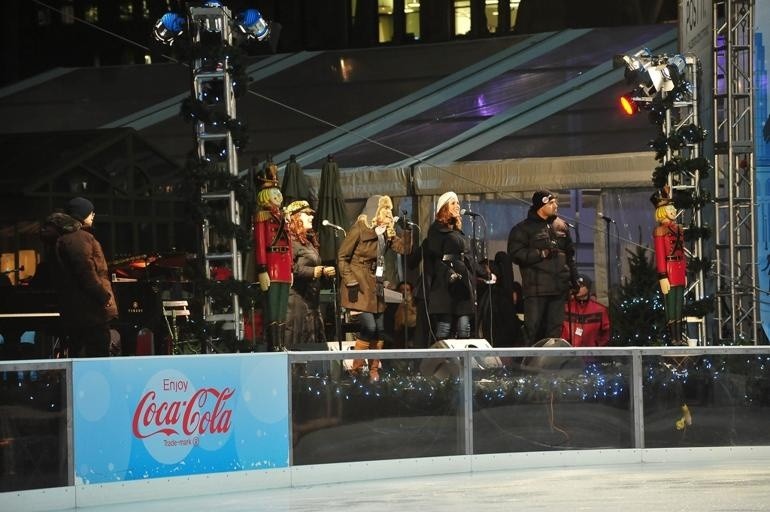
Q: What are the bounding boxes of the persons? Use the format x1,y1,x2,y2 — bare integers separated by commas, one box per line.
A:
40,196,119,357
255,187,294,352
653,201,689,346
283,189,609,382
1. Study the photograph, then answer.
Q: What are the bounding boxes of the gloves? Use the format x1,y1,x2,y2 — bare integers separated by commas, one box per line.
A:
397,216,411,230
348,284,363,302
448,272,462,282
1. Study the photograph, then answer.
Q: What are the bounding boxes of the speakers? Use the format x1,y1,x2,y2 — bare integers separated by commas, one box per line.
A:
520,336,585,380
418,337,504,380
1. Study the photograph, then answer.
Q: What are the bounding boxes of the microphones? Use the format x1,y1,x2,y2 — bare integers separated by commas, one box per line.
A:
322,220,342,230
597,212,616,224
393,216,414,226
459,208,481,218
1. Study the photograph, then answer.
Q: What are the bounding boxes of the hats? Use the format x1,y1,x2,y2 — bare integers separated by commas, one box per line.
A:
436,191,457,214
578,274,592,288
286,199,316,217
532,190,556,211
68,196,95,220
357,195,393,229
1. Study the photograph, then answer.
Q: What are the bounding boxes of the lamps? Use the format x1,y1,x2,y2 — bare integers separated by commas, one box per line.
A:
152,1,271,45
620,46,686,115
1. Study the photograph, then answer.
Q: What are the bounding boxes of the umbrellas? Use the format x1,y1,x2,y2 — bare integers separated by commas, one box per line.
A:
262,154,277,181
315,154,351,336
280,155,311,208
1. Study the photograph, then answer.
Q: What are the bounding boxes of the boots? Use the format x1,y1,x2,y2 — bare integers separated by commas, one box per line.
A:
369,340,384,384
352,336,371,375
